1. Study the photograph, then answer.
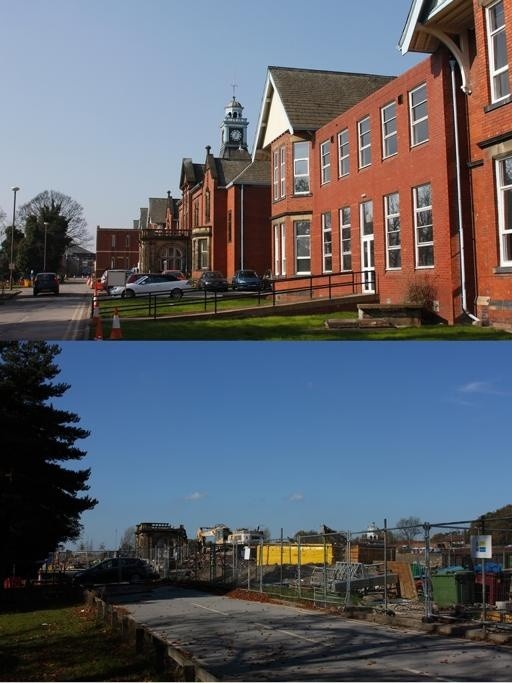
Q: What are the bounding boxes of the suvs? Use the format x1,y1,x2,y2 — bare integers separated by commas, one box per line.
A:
33,273,60,296
110,270,194,301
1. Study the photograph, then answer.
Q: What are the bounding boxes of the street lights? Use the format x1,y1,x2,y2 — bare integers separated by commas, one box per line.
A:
9,187,20,291
44,220,50,271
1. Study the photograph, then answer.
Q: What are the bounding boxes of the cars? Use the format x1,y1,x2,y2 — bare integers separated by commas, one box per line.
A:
198,271,228,292
73,558,159,588
232,269,261,290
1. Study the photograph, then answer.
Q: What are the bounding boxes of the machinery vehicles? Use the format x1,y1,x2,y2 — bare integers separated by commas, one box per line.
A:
197,525,264,557
320,524,391,545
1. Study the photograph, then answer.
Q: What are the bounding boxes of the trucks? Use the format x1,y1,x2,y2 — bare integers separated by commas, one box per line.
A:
101,269,134,287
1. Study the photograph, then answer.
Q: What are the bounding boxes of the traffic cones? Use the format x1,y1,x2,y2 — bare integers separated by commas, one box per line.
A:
108,305,124,339
90,287,107,341
